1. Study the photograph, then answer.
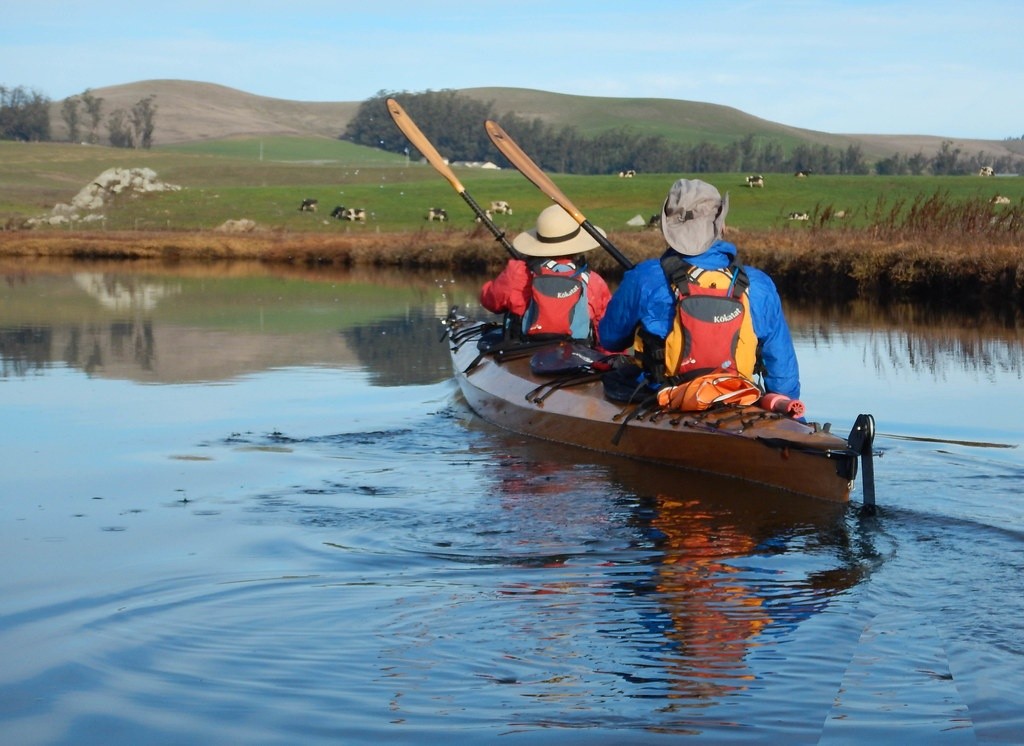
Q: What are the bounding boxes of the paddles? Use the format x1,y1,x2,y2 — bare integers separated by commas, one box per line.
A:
483,118,638,272
383,96,524,264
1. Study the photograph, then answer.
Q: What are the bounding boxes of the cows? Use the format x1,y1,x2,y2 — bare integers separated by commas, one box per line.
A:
425,207,448,222
789,212,809,221
618,169,636,178
331,206,366,221
300,199,318,212
978,167,995,177
794,170,810,178
746,175,764,187
474,201,512,222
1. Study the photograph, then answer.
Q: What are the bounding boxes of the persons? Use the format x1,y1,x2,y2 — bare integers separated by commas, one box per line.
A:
480,204,629,369
598,178,801,399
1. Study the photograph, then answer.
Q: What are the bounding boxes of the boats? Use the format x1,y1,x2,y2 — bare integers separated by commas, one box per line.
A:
441,303,877,508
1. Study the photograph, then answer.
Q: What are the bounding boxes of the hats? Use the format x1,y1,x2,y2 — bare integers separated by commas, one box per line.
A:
660,177,728,255
512,204,607,256
